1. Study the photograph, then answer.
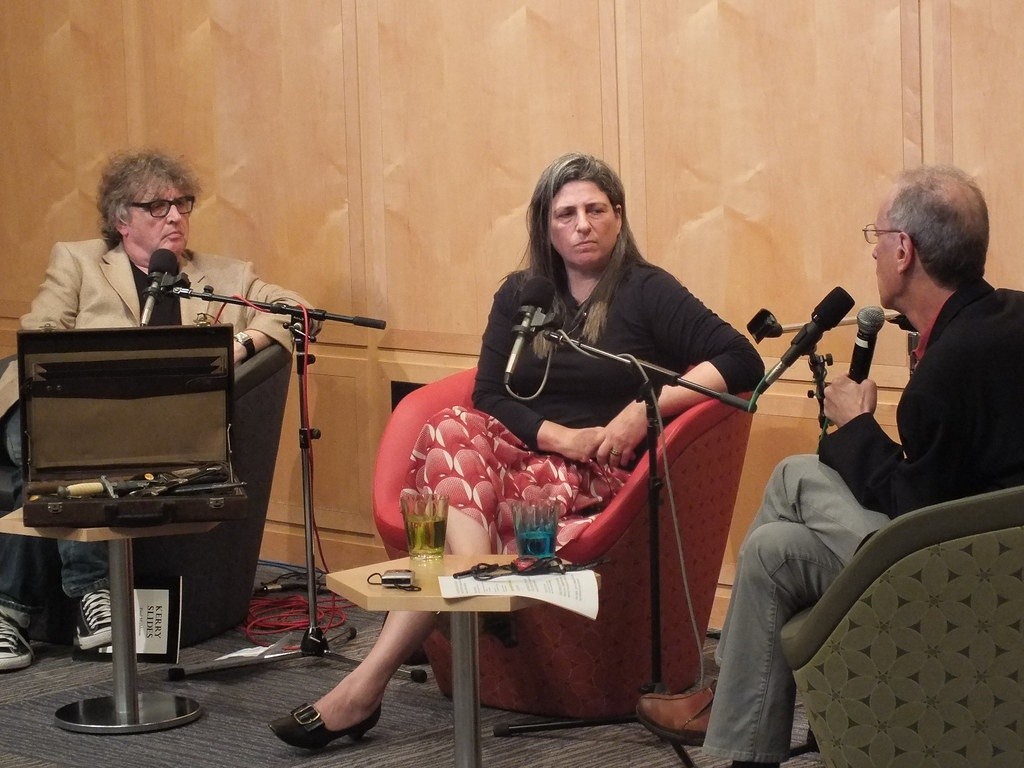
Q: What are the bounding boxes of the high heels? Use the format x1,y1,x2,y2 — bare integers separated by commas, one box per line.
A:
267,701,382,750
480,611,519,648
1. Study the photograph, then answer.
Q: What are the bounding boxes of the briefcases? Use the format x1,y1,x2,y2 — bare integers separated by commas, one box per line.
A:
17,322,249,528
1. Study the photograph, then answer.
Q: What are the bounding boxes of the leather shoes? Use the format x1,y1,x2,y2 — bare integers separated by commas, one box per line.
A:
636,675,716,747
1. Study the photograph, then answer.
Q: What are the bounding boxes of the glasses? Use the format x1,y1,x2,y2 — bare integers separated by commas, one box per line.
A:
862,224,917,247
127,195,194,218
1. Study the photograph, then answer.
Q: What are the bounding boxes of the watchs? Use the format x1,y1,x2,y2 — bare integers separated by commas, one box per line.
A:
234,332,255,363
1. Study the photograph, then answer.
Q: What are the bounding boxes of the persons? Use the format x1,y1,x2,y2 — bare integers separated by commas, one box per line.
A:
635,164,1024,768
267,153,766,751
0,150,324,670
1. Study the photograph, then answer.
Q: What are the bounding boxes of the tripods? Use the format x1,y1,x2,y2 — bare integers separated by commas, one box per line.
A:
489,316,761,768
159,273,430,682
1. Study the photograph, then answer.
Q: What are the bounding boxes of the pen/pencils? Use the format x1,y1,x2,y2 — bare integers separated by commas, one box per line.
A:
453,563,499,579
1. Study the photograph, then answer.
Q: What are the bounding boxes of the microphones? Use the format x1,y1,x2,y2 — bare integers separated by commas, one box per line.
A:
139,249,180,327
848,306,885,384
506,276,555,382
757,286,856,394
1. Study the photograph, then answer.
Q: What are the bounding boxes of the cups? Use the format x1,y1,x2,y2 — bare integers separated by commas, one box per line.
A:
510,497,561,559
400,493,449,559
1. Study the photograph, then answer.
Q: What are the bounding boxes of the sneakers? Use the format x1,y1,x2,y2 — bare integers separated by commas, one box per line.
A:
74,585,112,651
0,612,34,671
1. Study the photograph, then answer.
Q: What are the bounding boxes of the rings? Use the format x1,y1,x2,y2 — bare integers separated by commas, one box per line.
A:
611,449,620,456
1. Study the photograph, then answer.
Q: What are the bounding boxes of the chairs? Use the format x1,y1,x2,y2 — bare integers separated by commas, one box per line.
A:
780,486,1024,768
0,334,290,656
372,373,755,723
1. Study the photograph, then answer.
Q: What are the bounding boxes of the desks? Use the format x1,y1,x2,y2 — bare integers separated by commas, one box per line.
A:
0,504,219,735
327,555,600,766
